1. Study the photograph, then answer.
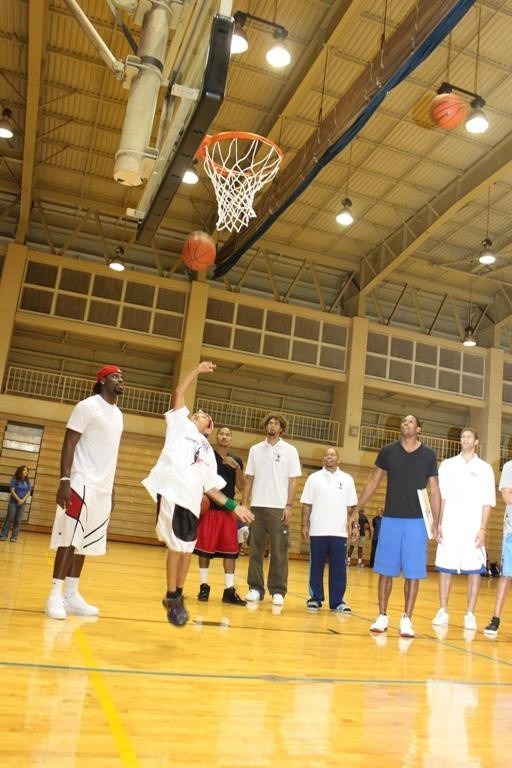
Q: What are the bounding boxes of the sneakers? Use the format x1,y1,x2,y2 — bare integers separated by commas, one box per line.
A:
272,593,284,605
369,614,388,632
62,593,99,616
398,637,413,652
10,536,17,542
400,613,415,637
0,535,7,541
463,629,475,644
198,584,210,601
433,610,448,626
485,618,500,633
463,614,476,630
433,625,447,642
222,587,246,606
371,633,386,646
246,589,261,601
48,595,66,619
166,597,188,625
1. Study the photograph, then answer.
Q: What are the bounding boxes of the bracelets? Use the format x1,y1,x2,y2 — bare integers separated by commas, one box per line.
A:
234,463,241,471
353,523,361,530
59,476,71,482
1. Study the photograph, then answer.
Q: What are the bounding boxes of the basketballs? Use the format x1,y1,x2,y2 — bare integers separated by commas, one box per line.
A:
430,94,466,132
181,231,217,272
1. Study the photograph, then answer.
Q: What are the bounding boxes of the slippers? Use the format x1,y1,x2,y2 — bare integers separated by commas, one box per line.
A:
307,601,319,611
330,604,351,614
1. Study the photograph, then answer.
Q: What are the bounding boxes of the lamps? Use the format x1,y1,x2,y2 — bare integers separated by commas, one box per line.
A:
0,108,14,139
230,12,249,54
266,27,291,69
109,247,126,272
335,141,354,226
181,163,200,185
463,265,477,347
478,185,495,265
466,96,489,134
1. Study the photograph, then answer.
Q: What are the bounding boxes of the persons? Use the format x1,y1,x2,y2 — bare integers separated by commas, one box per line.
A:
197,427,247,606
369,507,384,569
431,428,495,630
142,361,255,626
300,447,361,612
46,366,124,620
484,459,512,634
238,523,250,556
348,415,441,638
240,413,303,606
346,508,371,569
0,465,32,542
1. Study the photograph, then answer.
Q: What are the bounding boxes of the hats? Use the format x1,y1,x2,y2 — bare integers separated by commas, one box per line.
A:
92,366,121,394
